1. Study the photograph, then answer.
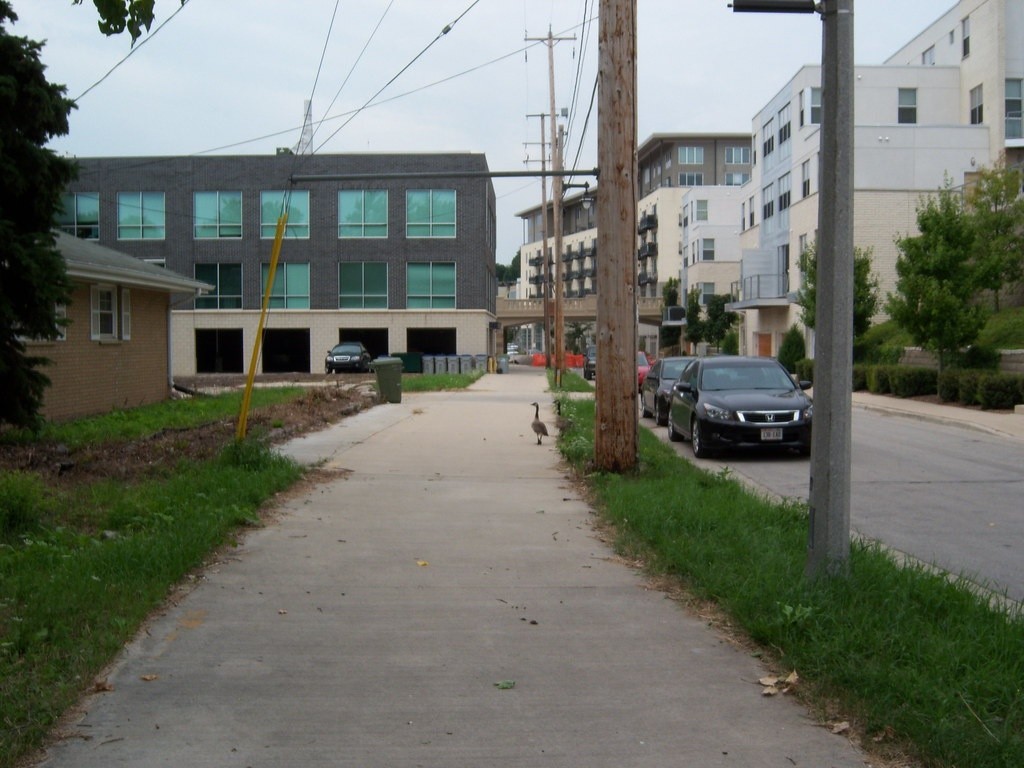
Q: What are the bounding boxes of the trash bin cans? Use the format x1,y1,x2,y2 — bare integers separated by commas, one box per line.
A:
476,355,487,373
373,358,403,404
447,355,460,375
435,354,447,376
460,355,472,376
422,354,434,375
497,354,510,374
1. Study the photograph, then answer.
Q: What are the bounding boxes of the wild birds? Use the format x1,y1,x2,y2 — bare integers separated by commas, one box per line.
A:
552,400,574,440
530,402,550,445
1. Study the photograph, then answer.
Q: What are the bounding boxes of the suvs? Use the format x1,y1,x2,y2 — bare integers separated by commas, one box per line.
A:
583,342,597,381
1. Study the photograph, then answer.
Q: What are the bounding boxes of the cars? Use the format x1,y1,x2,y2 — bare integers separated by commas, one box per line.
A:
637,351,656,391
668,356,812,459
638,356,702,428
326,341,373,375
